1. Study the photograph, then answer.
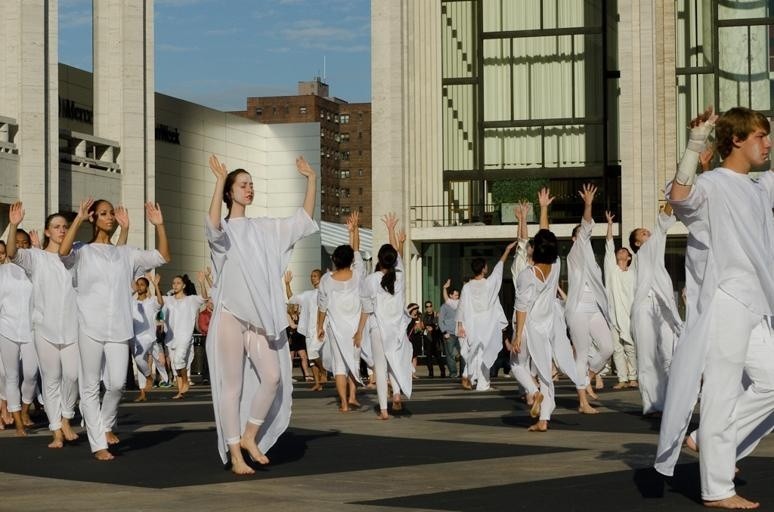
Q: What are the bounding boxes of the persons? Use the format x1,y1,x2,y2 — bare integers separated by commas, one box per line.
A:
201,154,320,474
284,211,417,420
443,183,687,431
0,196,171,461
406,289,461,380
647,141,753,483
127,268,216,401
671,102,773,510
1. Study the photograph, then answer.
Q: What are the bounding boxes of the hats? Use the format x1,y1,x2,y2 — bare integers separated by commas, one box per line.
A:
407,303,419,314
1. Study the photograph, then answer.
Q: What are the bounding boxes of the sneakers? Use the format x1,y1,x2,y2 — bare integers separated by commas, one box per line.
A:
159,380,172,388
